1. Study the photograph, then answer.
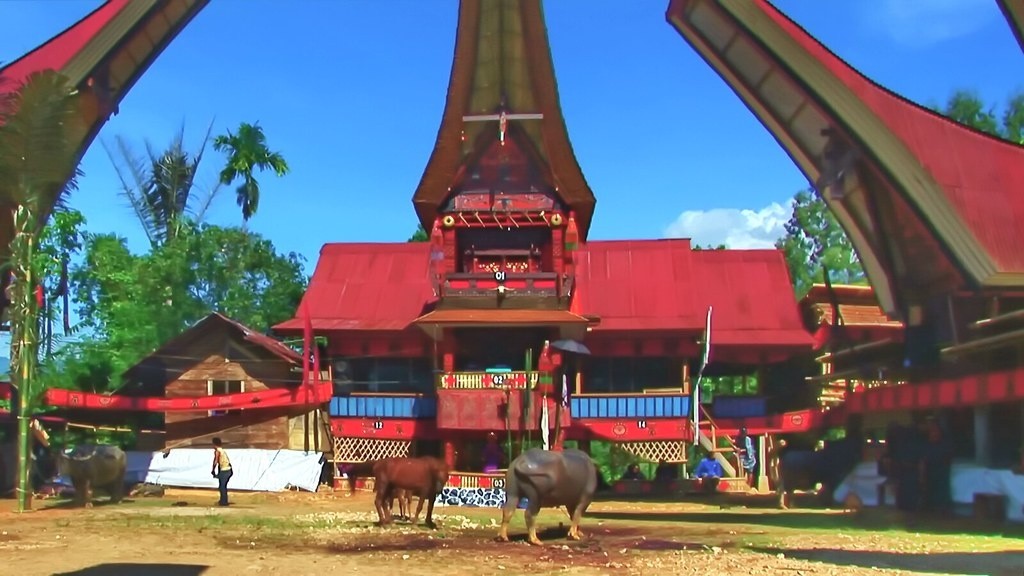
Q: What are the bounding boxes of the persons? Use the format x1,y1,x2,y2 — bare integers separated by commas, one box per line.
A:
538,340,562,371
735,426,756,486
623,463,646,480
211,437,233,506
695,451,724,498
655,459,673,481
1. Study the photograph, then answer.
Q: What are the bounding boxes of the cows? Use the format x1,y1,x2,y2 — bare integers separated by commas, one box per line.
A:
347,455,451,530
48,443,127,510
496,448,598,546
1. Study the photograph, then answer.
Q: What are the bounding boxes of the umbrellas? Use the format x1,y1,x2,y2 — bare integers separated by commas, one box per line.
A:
550,339,591,355
